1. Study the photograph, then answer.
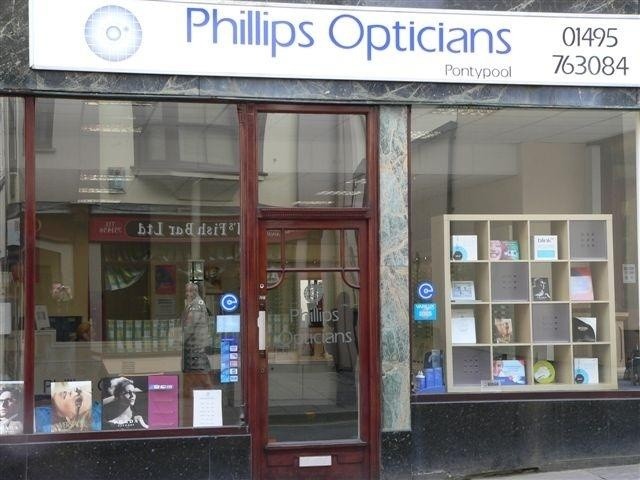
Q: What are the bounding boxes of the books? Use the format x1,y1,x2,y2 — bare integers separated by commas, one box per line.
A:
450,234,600,389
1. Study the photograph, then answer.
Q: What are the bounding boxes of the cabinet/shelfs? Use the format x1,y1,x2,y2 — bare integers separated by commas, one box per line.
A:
432,214,617,394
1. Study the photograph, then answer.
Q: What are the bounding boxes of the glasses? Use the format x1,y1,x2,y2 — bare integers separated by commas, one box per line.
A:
74,386,83,422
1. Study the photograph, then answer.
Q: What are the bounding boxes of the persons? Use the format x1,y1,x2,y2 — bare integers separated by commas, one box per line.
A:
103,379,148,429
52,383,93,434
183,282,213,372
0,387,25,435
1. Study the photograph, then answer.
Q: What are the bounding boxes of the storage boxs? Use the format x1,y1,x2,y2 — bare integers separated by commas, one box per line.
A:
574,356,600,385
494,357,526,388
450,234,479,261
534,233,558,260
571,316,598,342
